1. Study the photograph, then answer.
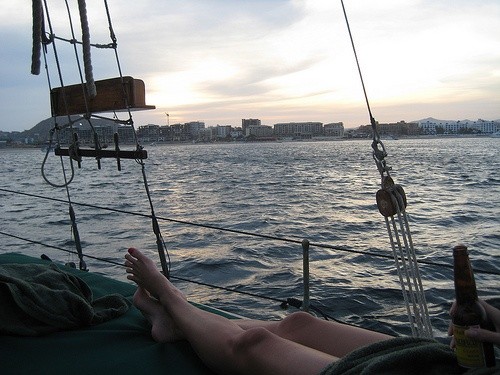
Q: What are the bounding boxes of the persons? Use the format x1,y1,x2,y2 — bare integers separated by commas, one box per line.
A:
124,245,500,375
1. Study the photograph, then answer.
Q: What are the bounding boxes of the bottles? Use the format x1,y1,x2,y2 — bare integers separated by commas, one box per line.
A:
452,245,496,375
